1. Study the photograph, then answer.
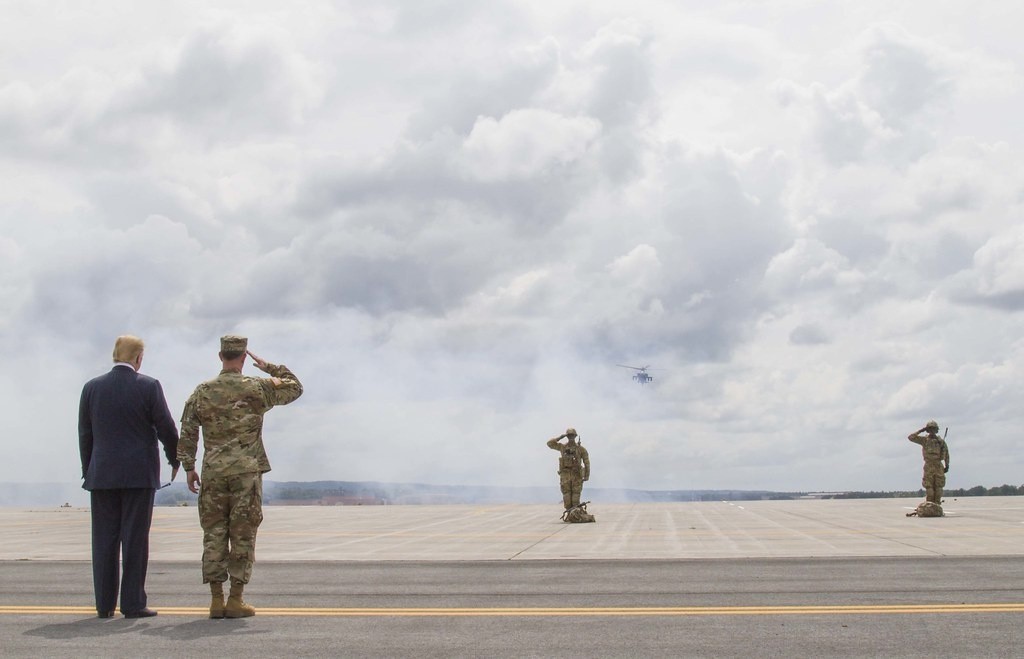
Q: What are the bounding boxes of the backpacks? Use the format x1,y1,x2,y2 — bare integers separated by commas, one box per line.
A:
917,501,943,517
564,506,596,522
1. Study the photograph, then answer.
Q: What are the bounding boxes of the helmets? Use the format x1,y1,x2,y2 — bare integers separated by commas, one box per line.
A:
925,420,939,433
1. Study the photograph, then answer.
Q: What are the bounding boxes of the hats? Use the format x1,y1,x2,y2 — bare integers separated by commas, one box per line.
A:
566,429,578,436
220,335,249,352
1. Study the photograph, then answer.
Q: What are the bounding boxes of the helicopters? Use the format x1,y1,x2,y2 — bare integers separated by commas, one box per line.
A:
617,364,659,384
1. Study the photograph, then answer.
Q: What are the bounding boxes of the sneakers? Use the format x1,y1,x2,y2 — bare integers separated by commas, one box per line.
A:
225,596,257,618
209,596,226,617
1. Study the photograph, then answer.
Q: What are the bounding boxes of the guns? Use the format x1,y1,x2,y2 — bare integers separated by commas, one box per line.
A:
578,435,581,445
560,500,591,524
905,499,945,517
943,427,949,440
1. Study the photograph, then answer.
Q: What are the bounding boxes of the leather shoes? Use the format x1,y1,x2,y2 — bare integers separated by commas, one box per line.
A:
125,607,158,618
96,610,115,618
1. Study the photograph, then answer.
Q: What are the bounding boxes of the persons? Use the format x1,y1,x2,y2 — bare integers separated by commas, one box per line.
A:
908,420,949,506
78,334,178,620
177,335,304,620
547,427,590,522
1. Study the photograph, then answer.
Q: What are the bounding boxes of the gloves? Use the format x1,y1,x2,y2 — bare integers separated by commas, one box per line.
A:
944,467,948,473
561,433,567,438
920,427,926,433
582,478,588,481
